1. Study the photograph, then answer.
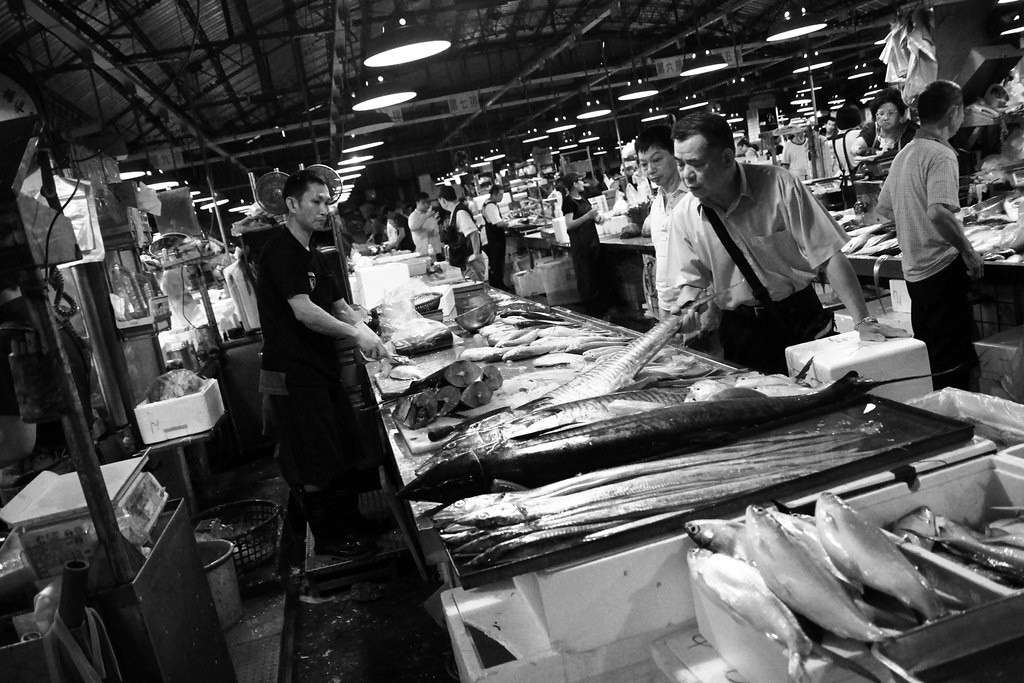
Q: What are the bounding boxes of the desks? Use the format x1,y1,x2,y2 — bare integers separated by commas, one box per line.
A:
342,224,1024,683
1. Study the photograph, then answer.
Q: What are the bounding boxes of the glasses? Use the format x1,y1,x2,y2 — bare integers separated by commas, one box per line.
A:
875,110,898,118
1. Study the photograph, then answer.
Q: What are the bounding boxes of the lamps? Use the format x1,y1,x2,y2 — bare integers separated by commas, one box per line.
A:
72,0,1024,218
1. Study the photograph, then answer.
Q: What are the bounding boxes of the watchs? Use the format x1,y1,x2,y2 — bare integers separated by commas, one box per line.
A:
853,315,878,330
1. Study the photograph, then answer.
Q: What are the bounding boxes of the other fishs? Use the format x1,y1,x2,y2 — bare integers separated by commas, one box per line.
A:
684,490,1024,683
395,276,988,569
961,200,1024,265
840,203,901,260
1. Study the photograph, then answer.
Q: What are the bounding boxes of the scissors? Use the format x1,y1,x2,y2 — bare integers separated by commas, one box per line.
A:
361,347,400,363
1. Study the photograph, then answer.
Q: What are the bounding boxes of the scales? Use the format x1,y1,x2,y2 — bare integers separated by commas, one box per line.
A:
0,447,169,575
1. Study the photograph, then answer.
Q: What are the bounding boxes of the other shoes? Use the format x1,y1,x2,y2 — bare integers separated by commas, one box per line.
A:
315,532,383,561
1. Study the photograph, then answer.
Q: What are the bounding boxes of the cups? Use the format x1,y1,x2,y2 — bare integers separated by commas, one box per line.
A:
180,345,200,373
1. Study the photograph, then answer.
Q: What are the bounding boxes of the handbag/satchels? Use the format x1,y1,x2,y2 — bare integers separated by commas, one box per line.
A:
791,309,834,344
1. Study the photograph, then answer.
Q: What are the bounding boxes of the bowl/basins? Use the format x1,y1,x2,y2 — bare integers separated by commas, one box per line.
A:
454,301,497,330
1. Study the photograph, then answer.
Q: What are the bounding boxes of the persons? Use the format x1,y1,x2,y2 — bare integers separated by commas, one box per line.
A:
736,139,783,159
464,182,477,198
523,168,634,204
664,111,912,382
344,186,481,274
820,116,837,140
256,169,392,562
824,103,867,177
561,173,607,320
481,185,512,291
780,120,814,184
850,89,921,180
875,79,985,393
634,126,690,341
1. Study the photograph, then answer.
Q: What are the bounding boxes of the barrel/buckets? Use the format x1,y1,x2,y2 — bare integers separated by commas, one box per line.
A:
197,540,242,629
97,424,138,462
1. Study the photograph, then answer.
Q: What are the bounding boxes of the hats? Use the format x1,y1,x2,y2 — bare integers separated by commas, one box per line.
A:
563,173,583,188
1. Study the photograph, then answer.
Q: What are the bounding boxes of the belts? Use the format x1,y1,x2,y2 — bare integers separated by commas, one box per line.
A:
723,283,814,320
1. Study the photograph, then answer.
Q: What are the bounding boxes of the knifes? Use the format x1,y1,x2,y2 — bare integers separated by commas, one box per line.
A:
429,405,509,441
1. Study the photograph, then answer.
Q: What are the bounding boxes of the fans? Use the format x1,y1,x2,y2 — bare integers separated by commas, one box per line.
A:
305,162,343,206
254,171,291,217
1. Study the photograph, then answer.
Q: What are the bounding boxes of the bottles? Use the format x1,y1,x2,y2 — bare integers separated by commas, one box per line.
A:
110,263,163,317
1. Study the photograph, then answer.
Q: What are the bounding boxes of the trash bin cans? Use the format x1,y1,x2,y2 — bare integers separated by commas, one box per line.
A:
537,257,581,307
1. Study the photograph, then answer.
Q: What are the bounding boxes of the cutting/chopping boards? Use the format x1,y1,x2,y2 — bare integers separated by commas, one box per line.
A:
391,368,574,454
372,360,452,400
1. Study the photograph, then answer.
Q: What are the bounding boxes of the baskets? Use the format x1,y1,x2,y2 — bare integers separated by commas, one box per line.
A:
368,292,443,319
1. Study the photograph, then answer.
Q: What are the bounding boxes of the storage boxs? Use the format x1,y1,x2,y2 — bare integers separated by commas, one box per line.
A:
958,185,969,197
959,196,968,208
971,280,1024,338
650,622,894,683
538,256,583,306
833,294,915,338
997,443,1024,464
888,279,912,313
840,453,1024,594
905,385,1024,453
133,377,227,445
552,216,571,244
437,584,568,683
785,330,934,403
453,279,485,294
609,214,628,234
513,268,546,297
970,196,1006,217
532,530,694,653
958,175,971,186
568,627,670,683
401,256,433,277
972,325,1024,382
